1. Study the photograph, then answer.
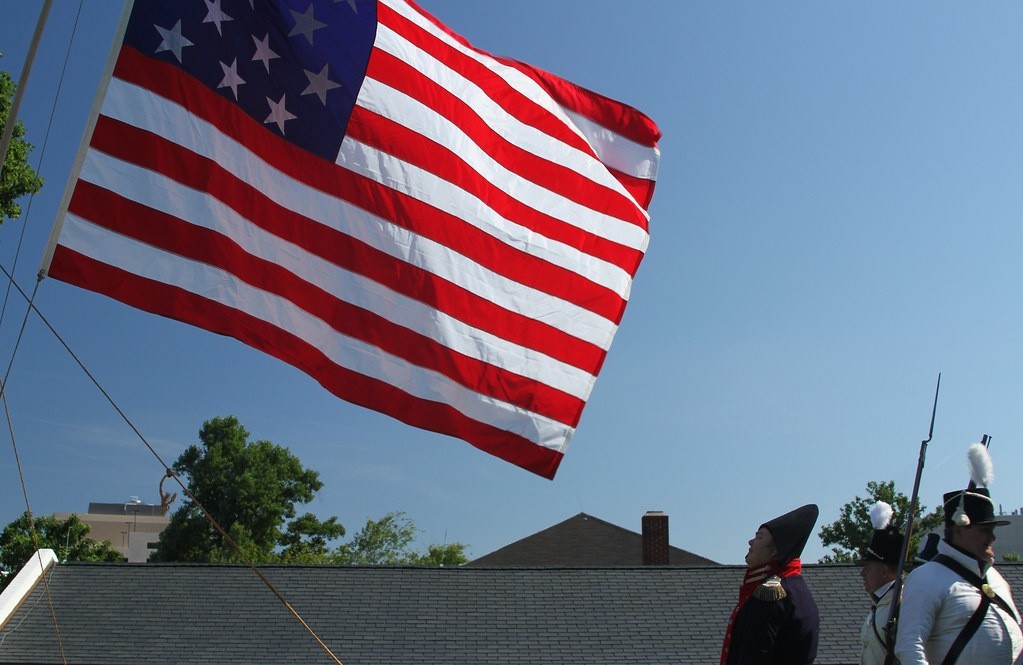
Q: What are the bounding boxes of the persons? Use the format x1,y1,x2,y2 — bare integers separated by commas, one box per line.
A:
720,504,821,665
859,525,906,665
894,489,1023,665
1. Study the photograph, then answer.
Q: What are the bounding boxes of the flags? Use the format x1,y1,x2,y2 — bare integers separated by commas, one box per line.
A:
46,0,661,482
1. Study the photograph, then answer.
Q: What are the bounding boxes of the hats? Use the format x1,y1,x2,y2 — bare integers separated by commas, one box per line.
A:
913,533,941,565
758,503,819,563
857,501,907,572
943,444,1011,527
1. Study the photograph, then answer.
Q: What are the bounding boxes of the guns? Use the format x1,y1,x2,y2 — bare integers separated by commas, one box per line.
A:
881,370,944,664
